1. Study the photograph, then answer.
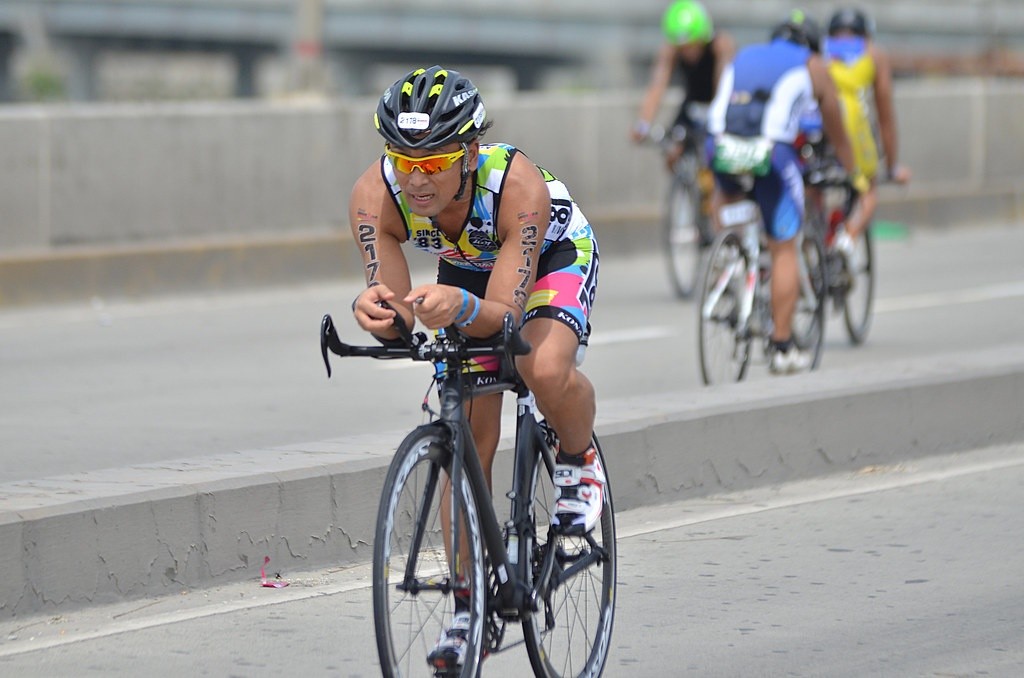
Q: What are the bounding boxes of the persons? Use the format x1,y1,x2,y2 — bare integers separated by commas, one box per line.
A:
628,0,908,375
346,63,603,678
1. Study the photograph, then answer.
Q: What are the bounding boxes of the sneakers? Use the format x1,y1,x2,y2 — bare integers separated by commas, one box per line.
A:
550,445,606,536
427,611,481,678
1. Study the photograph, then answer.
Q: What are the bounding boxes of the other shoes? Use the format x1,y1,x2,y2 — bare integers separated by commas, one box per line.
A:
772,347,810,374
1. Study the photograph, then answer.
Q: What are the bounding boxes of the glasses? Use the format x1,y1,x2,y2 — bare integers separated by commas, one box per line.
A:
385,142,469,175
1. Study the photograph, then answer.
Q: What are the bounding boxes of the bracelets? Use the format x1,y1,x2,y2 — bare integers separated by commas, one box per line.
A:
455,288,481,327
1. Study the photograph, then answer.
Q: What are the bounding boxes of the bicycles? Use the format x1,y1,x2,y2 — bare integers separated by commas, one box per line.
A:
321,298,618,678
638,120,895,384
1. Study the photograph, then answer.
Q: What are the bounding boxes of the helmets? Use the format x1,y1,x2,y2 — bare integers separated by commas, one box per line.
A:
373,65,487,150
829,7,866,36
662,1,712,45
771,9,819,53
